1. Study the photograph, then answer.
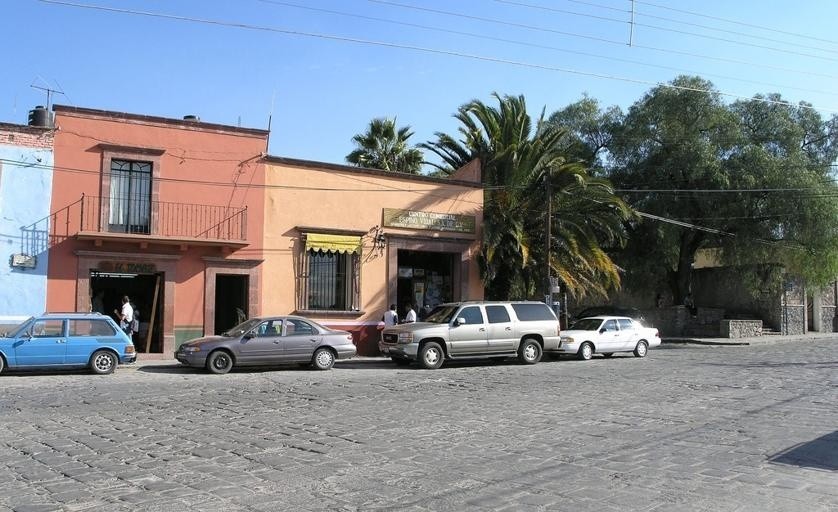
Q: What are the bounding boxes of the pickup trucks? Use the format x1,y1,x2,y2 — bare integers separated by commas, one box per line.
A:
379,301,565,370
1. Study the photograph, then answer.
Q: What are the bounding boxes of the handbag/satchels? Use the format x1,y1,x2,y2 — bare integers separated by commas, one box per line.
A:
130,319,138,332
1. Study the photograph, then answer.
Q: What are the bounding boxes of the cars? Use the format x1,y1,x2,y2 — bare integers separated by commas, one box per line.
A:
547,315,661,360
0,311,136,376
174,315,357,374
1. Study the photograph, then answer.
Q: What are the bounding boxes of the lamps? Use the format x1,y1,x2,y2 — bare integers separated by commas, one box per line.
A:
373,224,386,250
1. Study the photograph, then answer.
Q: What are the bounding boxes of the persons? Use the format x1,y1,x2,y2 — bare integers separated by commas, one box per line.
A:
235,304,247,326
683,293,697,319
381,304,398,329
92,289,106,315
113,296,140,343
402,303,416,323
